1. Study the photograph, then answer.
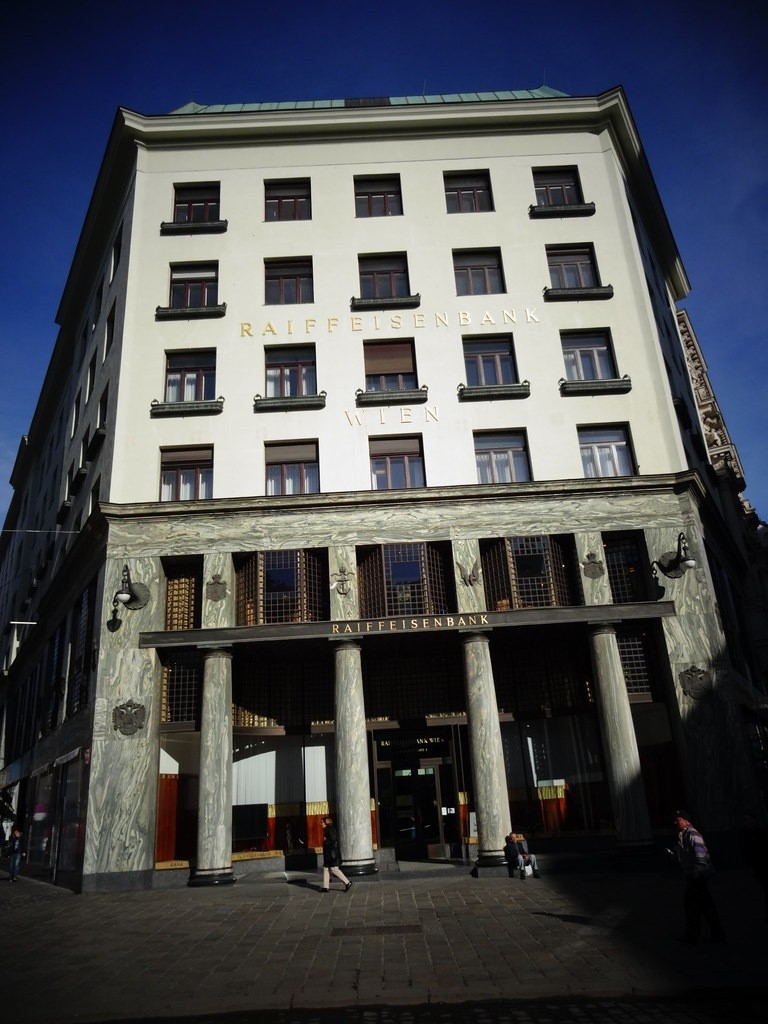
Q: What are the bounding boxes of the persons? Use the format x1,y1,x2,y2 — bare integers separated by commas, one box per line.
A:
319,817,352,892
507,832,541,880
7,827,27,882
672,812,727,944
737,813,768,924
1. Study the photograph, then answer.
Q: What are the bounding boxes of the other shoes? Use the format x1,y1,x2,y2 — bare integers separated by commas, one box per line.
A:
319,888,328,892
521,871,525,878
703,935,729,944
344,882,352,892
533,871,541,878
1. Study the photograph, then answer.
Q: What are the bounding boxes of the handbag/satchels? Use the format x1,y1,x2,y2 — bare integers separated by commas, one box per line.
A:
326,841,338,848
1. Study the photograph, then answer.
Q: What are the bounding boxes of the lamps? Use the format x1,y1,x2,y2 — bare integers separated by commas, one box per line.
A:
659,533,698,580
116,565,151,610
